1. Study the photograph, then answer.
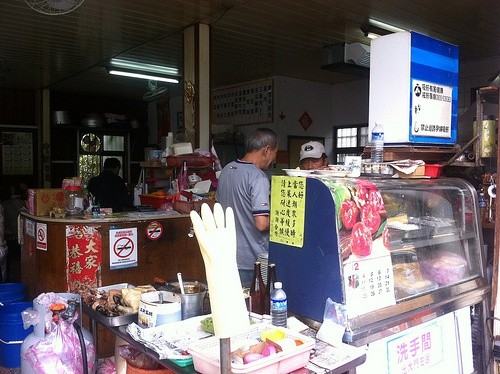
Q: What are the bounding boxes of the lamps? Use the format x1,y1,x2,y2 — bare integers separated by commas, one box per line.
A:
360,17,406,41
105,59,180,84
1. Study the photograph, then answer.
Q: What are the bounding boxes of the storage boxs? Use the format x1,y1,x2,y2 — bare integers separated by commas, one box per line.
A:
397,162,442,178
138,156,216,215
186,323,317,374
78,283,144,327
26,186,81,216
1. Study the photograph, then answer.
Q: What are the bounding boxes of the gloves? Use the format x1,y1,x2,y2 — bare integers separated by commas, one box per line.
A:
189,202,250,340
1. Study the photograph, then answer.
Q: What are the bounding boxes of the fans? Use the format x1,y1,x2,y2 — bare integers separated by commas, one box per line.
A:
142,82,169,101
25,0,86,16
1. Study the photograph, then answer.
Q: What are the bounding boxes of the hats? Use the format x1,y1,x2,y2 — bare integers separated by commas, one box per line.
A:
298,141,325,162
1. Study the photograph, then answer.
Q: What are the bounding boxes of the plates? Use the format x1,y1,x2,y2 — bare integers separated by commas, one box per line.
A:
282,169,314,177
315,164,351,178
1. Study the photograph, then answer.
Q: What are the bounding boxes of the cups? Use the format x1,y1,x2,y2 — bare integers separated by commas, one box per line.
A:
344,156,362,178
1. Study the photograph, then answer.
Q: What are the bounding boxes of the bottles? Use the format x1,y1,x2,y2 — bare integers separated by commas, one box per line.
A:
488,182,496,223
83,185,100,216
63,185,84,216
250,261,266,315
478,188,488,223
371,120,384,163
270,281,288,329
265,263,278,315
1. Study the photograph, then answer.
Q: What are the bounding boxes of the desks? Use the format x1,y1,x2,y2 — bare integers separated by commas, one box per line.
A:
79,284,372,374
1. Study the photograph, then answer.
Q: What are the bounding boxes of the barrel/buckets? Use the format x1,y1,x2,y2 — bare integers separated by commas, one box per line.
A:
171,283,207,320
0,301,33,368
138,291,182,326
0,283,27,306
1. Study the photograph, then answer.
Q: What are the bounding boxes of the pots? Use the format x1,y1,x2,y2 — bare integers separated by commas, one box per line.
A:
144,147,162,162
52,110,72,127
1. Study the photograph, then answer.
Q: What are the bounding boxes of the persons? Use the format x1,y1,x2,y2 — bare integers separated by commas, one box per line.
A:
216,128,279,289
300,141,328,169
88,158,131,210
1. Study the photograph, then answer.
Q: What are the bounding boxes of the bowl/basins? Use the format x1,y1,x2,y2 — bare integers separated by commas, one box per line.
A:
421,249,468,287
